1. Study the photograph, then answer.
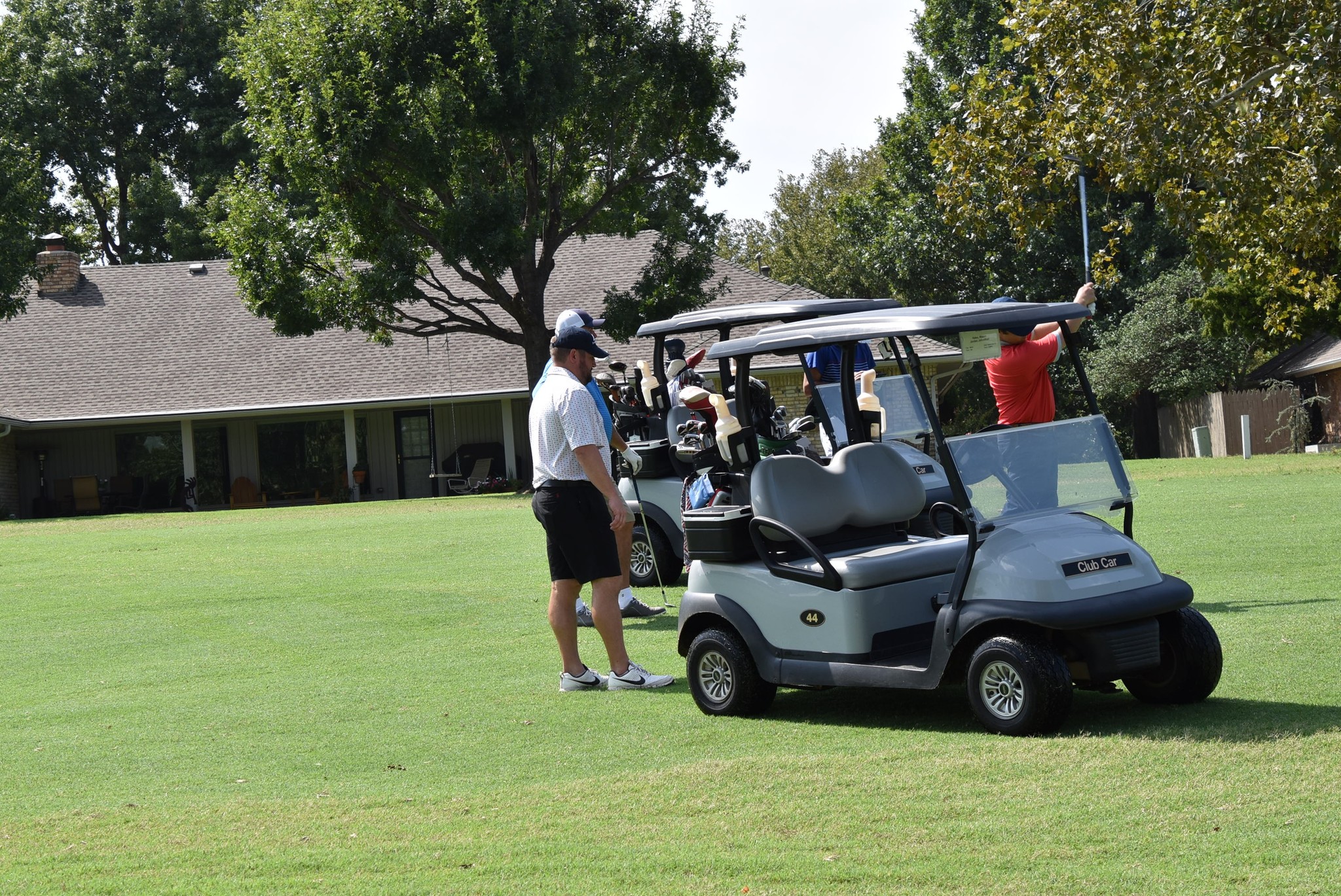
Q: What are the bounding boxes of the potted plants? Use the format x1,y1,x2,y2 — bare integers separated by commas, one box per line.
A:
352,463,366,484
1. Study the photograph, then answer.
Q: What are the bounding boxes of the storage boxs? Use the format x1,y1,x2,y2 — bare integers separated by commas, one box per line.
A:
617,439,671,478
683,505,753,560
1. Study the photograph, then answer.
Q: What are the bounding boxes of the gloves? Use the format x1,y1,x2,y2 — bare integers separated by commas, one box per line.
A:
620,444,642,475
1082,296,1097,325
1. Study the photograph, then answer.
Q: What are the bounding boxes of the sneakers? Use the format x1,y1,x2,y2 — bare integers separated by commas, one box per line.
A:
576,602,594,627
559,663,609,692
620,595,666,619
608,659,674,691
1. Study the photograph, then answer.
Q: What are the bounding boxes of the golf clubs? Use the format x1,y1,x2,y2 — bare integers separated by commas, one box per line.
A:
676,423,690,437
768,405,817,441
609,361,637,406
690,412,706,449
683,434,703,450
632,417,647,440
685,420,706,450
626,459,678,609
698,422,712,446
699,433,706,448
1062,154,1093,322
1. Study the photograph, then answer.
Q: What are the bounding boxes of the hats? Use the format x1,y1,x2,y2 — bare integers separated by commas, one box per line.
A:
555,308,606,337
992,297,1037,336
552,326,610,358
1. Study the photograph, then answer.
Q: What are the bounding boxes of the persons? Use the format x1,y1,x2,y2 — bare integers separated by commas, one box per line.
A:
533,308,665,627
529,329,676,693
803,341,877,456
983,282,1096,515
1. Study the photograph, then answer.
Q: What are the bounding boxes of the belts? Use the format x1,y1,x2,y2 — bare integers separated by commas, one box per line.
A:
539,479,591,488
1012,420,1056,432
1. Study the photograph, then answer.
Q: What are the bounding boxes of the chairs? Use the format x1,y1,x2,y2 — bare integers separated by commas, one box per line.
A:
448,458,492,496
315,469,349,505
51,473,151,517
226,476,269,508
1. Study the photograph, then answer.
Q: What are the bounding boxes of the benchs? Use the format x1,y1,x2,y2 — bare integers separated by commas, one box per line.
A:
667,398,739,481
749,443,970,590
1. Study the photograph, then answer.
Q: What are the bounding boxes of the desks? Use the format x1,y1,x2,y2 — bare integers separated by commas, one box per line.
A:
280,491,306,506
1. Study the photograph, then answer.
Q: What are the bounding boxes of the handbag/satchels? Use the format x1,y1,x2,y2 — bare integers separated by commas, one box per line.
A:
688,472,715,510
